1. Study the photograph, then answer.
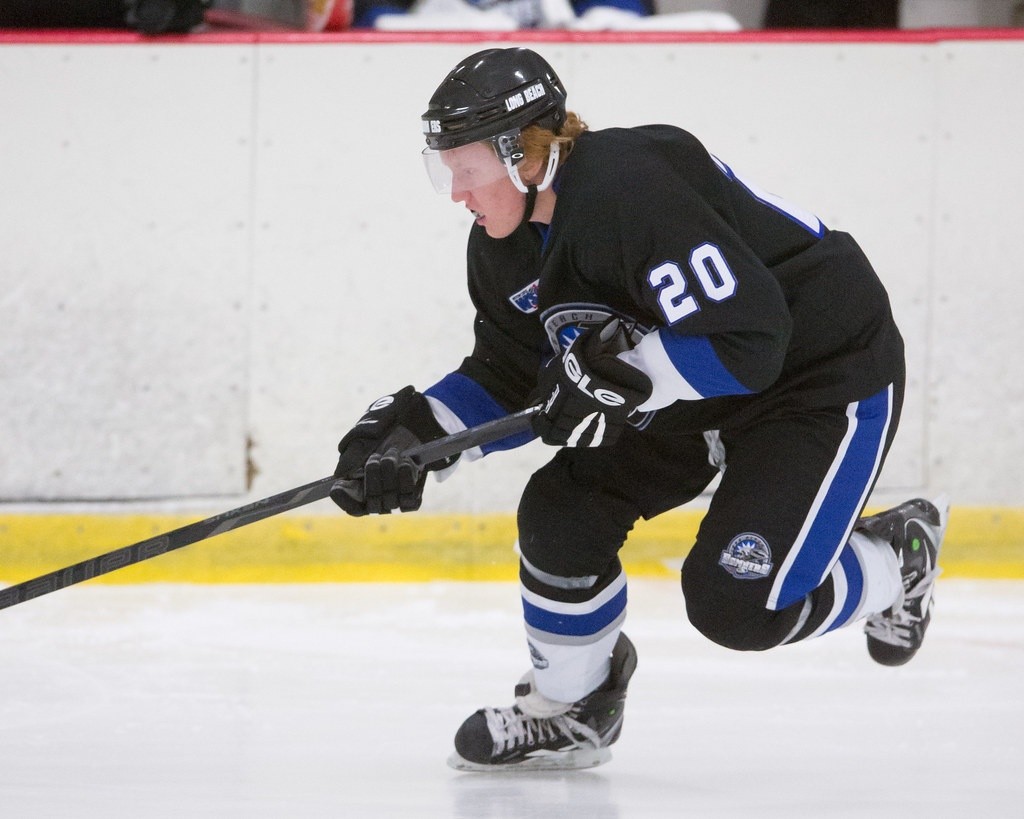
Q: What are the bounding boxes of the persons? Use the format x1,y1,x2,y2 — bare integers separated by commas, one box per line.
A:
329,46,951,772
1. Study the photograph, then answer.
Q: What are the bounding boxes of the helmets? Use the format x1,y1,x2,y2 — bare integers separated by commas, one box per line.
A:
420,48,567,166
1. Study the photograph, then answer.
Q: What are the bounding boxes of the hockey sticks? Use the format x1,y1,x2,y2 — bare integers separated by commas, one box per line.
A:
0,402,553,612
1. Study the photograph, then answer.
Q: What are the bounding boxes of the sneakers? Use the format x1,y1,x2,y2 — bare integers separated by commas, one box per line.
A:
447,633,637,768
856,492,949,668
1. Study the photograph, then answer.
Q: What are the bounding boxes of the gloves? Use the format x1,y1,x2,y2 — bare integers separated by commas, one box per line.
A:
329,385,461,518
524,316,652,449
